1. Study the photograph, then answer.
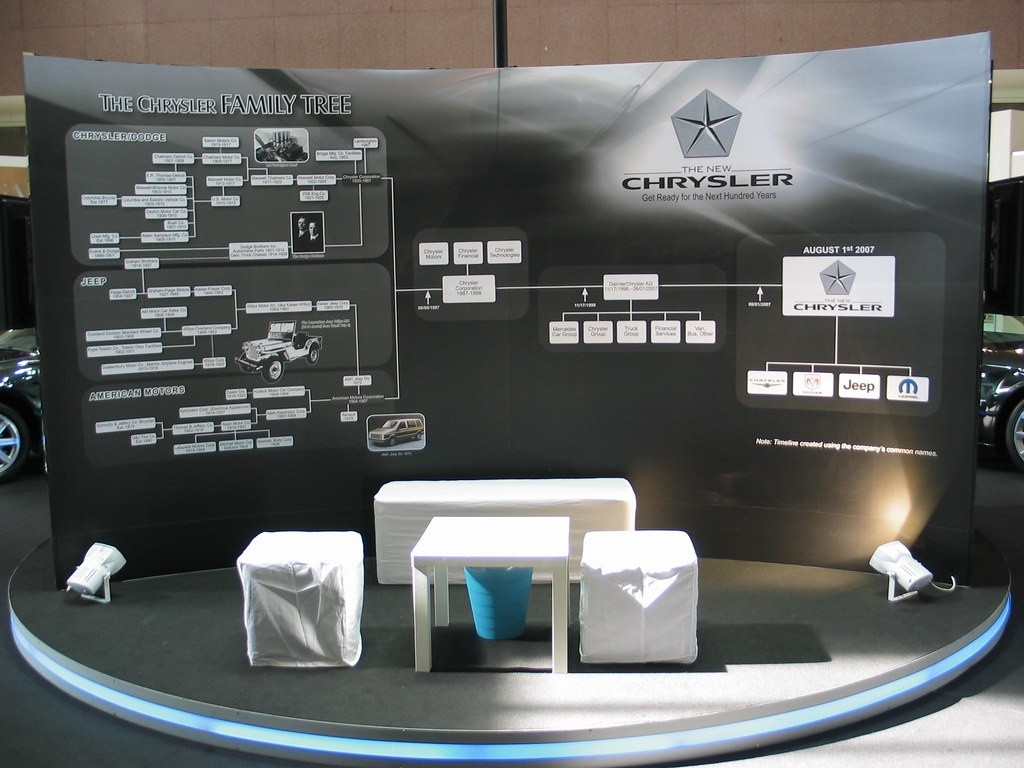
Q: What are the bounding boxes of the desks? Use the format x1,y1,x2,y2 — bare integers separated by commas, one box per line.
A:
411,517,571,674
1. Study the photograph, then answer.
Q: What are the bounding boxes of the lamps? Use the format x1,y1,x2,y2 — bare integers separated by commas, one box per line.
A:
66,542,126,604
869,540,957,603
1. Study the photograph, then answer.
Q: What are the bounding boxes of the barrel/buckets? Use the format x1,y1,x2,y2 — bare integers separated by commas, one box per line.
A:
465,568,534,639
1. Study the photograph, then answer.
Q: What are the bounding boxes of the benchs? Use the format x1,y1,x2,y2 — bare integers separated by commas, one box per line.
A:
373,478,637,585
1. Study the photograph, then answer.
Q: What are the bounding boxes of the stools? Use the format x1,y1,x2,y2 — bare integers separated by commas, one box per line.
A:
579,530,698,664
236,531,365,668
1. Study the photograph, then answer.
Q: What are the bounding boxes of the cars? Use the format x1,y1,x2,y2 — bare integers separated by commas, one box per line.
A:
0,328,43,488
982,338,1024,475
